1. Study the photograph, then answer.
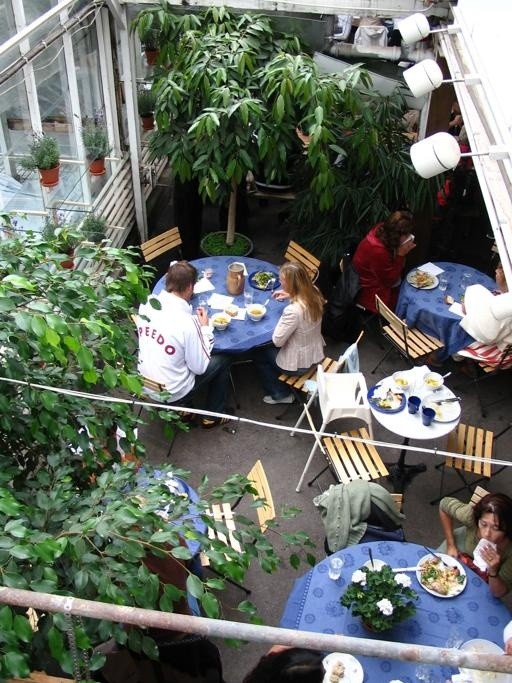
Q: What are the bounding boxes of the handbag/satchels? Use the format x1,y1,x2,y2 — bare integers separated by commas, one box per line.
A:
457,550,489,584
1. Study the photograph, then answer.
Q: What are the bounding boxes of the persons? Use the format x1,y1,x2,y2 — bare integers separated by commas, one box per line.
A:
256,261,326,405
352,209,417,319
438,493,512,617
430,261,512,377
137,260,236,429
239,643,326,683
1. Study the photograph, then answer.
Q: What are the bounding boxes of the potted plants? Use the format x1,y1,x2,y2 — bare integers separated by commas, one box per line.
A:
42,214,81,270
73,114,114,176
140,29,161,66
137,84,158,131
128,4,385,257
18,130,62,187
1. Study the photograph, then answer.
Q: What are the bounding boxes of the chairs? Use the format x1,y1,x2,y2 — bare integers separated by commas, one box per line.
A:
453,342,512,419
140,227,184,263
303,403,390,496
131,376,201,458
491,241,499,259
284,240,322,283
199,459,276,596
428,424,510,507
467,485,492,508
371,294,445,374
274,330,365,421
388,493,404,514
339,258,387,351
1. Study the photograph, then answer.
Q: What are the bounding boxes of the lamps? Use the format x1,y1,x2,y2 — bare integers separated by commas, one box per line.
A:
409,131,511,179
402,59,481,99
397,13,461,44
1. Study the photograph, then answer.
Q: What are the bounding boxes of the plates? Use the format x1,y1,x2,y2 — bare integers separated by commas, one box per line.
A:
458,639,504,680
406,269,440,291
422,390,462,423
165,476,189,513
416,552,468,598
322,651,364,683
248,270,282,291
366,384,406,415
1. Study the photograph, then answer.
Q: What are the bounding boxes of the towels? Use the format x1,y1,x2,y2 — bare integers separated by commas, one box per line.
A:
417,262,446,278
401,233,415,247
191,278,216,294
451,674,468,683
232,261,249,278
222,307,247,321
448,301,466,318
207,292,234,311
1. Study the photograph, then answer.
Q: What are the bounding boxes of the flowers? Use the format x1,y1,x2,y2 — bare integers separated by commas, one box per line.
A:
339,564,419,634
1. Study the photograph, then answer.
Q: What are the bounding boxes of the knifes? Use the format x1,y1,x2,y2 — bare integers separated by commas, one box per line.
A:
432,397,460,404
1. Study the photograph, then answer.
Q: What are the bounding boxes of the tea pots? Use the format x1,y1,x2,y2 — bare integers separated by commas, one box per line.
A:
225,262,246,296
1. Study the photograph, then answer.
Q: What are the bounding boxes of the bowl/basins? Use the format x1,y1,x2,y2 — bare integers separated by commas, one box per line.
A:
361,559,386,572
246,303,267,323
210,312,232,331
392,370,411,390
422,371,445,391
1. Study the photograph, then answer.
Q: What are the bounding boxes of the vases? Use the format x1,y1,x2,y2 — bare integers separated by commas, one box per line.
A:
361,621,373,635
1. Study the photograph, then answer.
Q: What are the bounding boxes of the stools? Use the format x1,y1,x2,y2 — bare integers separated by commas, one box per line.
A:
290,364,375,493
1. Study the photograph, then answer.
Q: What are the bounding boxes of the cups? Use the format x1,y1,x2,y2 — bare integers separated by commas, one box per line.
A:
328,556,343,579
244,287,254,308
444,626,464,650
416,662,430,680
199,295,208,316
440,271,452,292
408,395,421,415
461,269,473,291
421,407,436,426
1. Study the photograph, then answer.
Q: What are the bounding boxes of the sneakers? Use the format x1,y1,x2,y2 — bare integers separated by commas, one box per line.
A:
199,406,235,430
262,394,297,406
178,412,199,428
